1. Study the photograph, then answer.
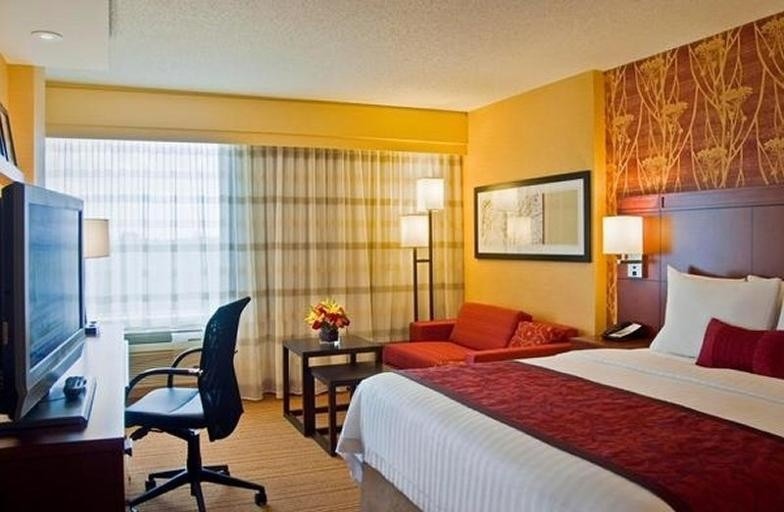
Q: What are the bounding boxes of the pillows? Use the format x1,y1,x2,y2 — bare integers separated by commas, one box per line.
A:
695,318,783,380
648,264,781,361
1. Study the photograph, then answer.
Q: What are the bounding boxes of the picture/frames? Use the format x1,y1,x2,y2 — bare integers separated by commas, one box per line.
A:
474,169,590,263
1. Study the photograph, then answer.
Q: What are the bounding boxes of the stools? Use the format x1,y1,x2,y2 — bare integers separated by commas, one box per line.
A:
313,363,381,457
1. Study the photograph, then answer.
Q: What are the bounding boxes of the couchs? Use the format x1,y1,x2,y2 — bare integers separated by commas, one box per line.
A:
382,303,578,367
126,296,269,511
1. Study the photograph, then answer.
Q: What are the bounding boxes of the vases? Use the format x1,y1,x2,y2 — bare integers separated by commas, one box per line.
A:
319,322,342,347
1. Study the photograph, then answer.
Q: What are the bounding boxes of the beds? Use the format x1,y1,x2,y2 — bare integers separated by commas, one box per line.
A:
335,182,784,512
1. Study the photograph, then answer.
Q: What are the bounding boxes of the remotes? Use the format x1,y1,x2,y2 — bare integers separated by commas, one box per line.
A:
63,376,87,397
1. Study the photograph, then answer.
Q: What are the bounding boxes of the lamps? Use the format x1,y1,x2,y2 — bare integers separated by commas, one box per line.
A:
416,177,445,321
602,216,644,279
82,219,109,335
400,215,430,322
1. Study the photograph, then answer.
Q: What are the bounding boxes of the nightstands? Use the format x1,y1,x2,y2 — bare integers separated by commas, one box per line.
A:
569,331,648,351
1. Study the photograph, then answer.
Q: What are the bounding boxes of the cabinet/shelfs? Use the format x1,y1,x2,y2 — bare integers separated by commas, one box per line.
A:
0,336,127,511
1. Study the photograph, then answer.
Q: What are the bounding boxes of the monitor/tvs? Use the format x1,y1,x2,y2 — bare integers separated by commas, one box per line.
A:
0,182,97,431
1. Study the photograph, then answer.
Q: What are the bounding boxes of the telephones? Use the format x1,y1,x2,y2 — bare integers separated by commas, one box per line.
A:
600,321,643,341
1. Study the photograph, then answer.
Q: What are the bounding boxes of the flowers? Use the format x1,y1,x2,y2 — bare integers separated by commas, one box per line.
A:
303,295,349,330
